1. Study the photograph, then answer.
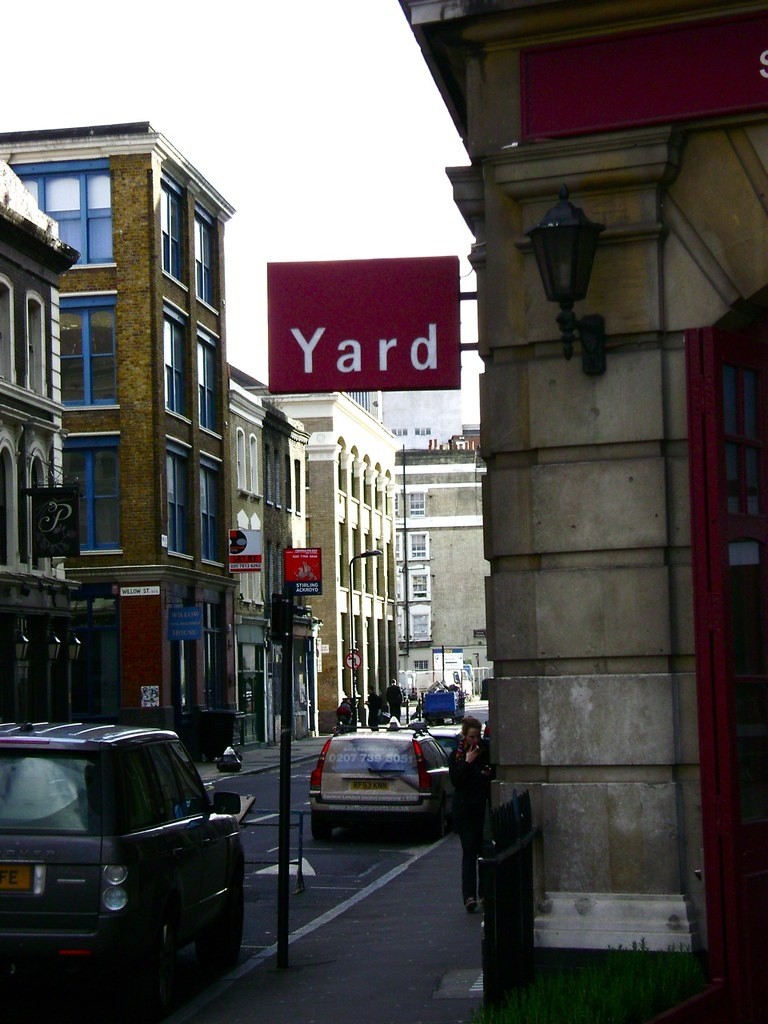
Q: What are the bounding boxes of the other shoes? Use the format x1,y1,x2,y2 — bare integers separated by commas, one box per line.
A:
465,897,477,913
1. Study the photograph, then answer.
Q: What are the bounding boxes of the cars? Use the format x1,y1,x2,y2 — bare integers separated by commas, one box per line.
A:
309,716,453,843
400,722,486,758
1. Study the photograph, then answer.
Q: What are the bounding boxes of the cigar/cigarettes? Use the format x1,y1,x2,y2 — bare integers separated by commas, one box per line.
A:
476,745,479,749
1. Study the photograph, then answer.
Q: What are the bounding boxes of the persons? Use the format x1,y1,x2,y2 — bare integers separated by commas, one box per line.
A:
386,679,404,723
448,717,490,912
365,685,379,731
337,693,356,733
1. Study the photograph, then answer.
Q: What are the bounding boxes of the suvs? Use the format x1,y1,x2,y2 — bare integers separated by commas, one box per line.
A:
0,717,249,1022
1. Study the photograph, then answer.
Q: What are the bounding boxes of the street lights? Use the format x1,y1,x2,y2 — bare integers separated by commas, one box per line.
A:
348,549,385,728
